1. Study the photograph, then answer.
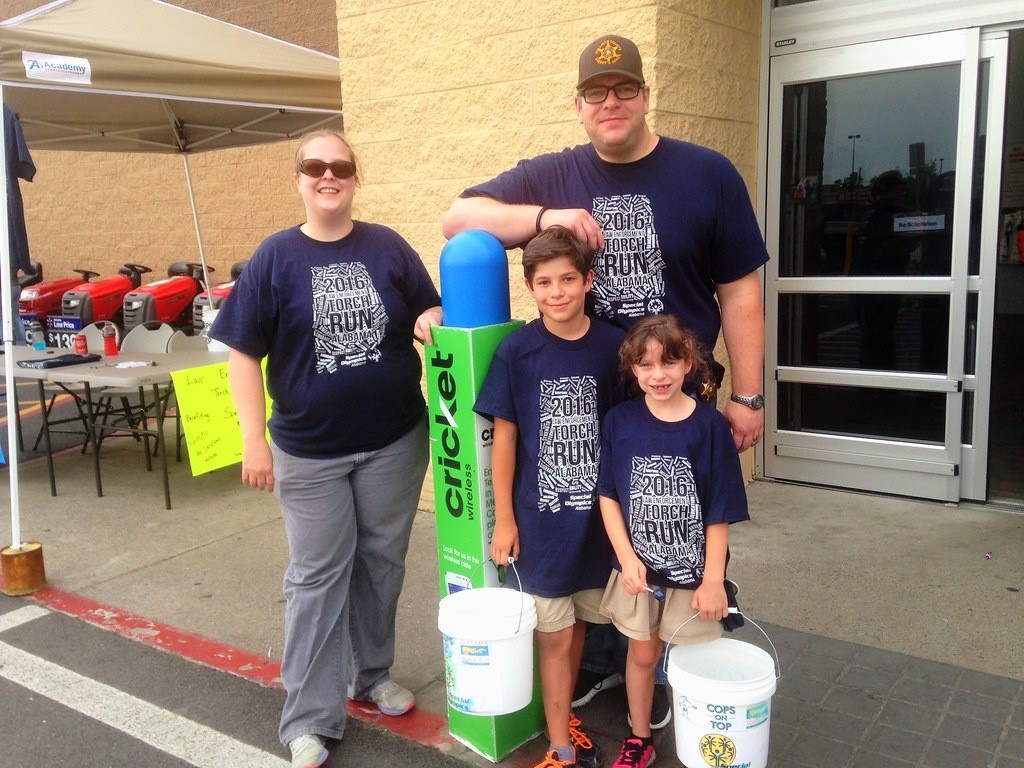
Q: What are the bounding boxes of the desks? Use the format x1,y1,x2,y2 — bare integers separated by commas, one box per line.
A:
0,343,104,497
47,352,229,511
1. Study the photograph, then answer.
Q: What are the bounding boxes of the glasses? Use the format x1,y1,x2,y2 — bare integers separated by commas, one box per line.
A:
298,159,356,178
581,82,642,104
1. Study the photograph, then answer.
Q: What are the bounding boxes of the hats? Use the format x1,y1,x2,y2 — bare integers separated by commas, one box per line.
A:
577,34,645,90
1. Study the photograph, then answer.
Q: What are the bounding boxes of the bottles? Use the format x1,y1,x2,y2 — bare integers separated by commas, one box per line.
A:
102,323,118,355
30,318,45,350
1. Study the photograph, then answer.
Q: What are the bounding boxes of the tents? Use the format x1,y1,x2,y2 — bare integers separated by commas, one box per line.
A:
0,0,344,549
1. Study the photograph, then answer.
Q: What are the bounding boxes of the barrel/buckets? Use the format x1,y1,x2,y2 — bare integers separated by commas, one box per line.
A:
662,607,781,768
438,556,537,716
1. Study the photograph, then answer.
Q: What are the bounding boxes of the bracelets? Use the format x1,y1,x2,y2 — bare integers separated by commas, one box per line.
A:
536,207,552,233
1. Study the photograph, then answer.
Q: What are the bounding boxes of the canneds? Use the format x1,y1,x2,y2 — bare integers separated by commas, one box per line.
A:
74,334,88,355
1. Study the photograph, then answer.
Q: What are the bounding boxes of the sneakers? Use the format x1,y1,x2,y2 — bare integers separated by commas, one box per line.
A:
543,712,604,768
627,685,671,729
534,746,576,768
571,670,625,707
356,678,416,716
611,738,656,768
288,733,329,768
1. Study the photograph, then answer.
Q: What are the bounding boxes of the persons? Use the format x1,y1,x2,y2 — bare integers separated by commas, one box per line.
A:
597,314,751,768
442,35,772,729
207,130,443,768
473,224,643,768
852,172,953,401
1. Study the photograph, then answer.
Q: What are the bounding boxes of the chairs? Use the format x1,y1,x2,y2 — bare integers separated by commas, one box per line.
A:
166,327,214,476
93,321,175,472
33,320,119,454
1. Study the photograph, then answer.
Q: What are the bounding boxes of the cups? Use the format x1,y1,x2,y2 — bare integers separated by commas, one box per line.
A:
75,334,87,353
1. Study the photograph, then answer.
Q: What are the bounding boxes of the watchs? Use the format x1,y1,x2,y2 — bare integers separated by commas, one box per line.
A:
731,394,764,409
